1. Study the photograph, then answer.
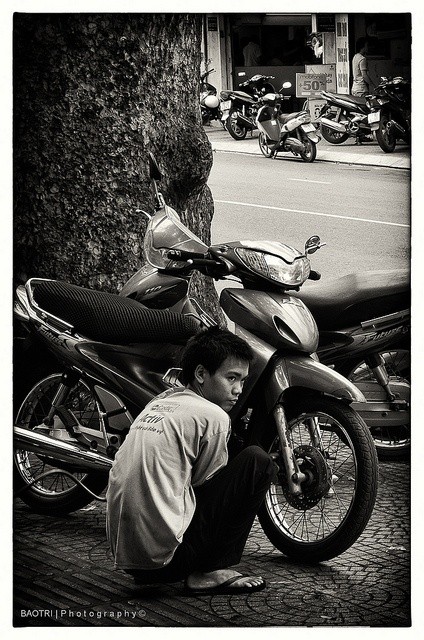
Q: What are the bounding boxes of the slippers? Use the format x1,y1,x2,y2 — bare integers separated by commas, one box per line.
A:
184,572,265,596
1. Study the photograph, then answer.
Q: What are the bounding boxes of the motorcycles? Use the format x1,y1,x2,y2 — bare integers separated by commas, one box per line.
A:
220,70,293,141
200,59,227,134
14,235,380,564
318,76,401,144
125,150,411,462
250,84,322,163
366,75,411,153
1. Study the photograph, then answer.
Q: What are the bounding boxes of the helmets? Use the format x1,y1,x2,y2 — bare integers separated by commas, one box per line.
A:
203,95,220,109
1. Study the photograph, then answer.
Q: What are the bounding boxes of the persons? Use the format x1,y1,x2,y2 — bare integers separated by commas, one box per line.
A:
243,34,263,67
351,41,381,142
105,325,276,597
